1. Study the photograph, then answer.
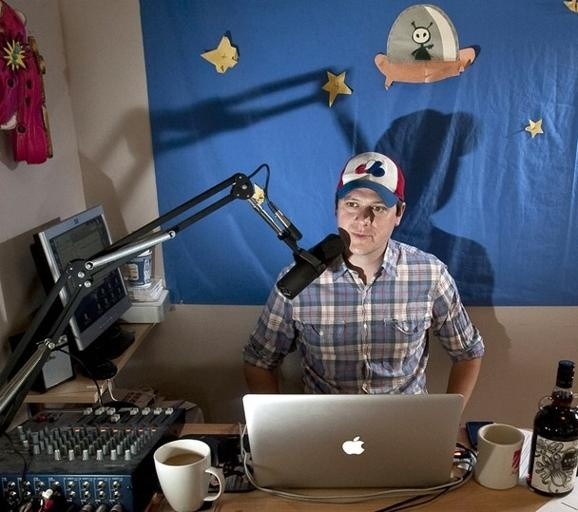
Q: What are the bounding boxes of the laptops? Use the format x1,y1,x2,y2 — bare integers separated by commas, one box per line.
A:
240,393,466,493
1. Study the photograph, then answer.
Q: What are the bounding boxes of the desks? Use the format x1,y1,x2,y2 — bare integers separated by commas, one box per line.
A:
23,323,157,416
148,424,553,512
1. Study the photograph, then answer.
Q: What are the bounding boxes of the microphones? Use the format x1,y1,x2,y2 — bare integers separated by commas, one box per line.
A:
275,232,347,302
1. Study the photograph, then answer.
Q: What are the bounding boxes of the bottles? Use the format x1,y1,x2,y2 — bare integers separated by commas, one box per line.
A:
527,360,578,499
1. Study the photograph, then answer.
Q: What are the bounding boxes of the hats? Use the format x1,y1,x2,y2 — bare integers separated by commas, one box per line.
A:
336,152,406,207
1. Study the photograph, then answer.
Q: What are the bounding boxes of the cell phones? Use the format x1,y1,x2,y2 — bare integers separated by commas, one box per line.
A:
465,420,495,448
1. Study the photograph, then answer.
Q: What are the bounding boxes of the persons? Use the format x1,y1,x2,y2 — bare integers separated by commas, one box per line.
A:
242,151,484,413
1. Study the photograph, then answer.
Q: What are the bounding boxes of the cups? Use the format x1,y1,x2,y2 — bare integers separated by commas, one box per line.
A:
155,439,226,512
475,423,525,490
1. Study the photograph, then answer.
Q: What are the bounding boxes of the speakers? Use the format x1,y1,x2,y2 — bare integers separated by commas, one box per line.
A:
35,333,74,392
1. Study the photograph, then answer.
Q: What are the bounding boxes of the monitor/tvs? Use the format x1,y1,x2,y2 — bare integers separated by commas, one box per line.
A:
33,201,136,380
